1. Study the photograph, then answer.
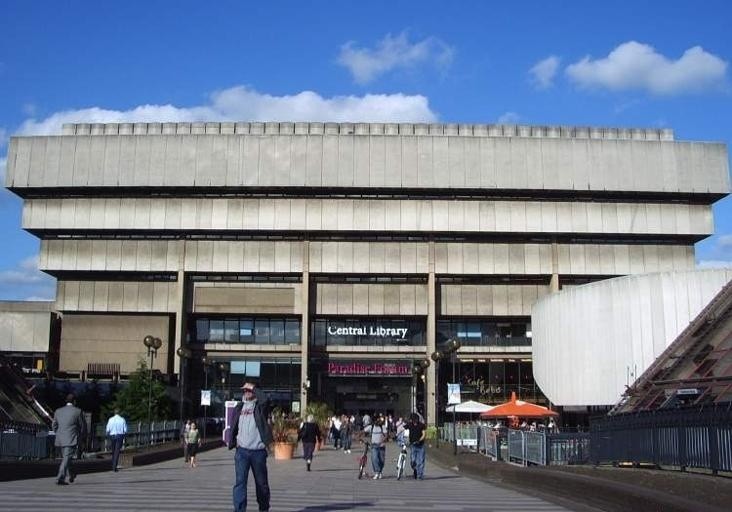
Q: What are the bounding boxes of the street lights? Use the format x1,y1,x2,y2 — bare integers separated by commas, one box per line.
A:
177,346,191,440
144,335,162,453
432,351,442,448
303,377,312,407
218,362,230,429
445,336,462,456
412,358,429,442
202,356,213,442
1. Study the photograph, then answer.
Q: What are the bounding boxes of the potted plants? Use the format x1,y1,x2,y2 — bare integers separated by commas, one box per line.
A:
267,406,301,461
303,402,334,450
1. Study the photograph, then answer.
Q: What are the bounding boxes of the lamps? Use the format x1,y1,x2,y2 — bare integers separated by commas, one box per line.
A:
302,378,311,391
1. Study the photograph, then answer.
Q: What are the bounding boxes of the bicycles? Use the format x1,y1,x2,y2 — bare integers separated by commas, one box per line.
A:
357,439,378,479
393,438,418,480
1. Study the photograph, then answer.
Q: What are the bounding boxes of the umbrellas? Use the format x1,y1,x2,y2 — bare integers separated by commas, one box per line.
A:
445,400,495,414
479,392,560,417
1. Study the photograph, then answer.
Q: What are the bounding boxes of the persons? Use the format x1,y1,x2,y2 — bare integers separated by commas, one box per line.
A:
106,408,127,472
227,383,271,512
52,394,87,484
179,393,427,487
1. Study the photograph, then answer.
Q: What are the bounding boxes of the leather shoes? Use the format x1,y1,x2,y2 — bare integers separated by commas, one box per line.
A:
56,479,69,485
